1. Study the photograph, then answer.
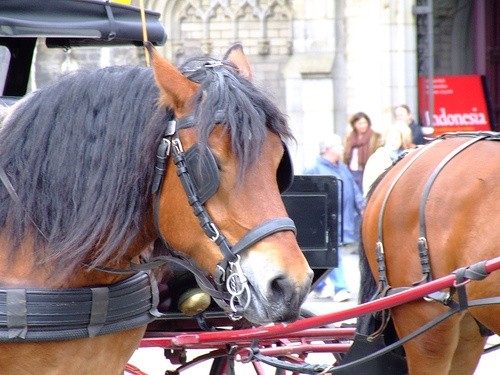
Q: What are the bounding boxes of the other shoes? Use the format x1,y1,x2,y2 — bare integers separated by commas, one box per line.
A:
311,292,332,301
333,289,351,302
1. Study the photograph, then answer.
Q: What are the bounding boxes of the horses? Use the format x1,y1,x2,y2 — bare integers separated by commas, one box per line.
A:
354,132,499,375
1,39,315,375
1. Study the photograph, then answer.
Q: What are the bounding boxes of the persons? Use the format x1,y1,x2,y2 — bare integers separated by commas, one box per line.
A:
362,102,424,201
302,133,364,303
342,112,384,255
